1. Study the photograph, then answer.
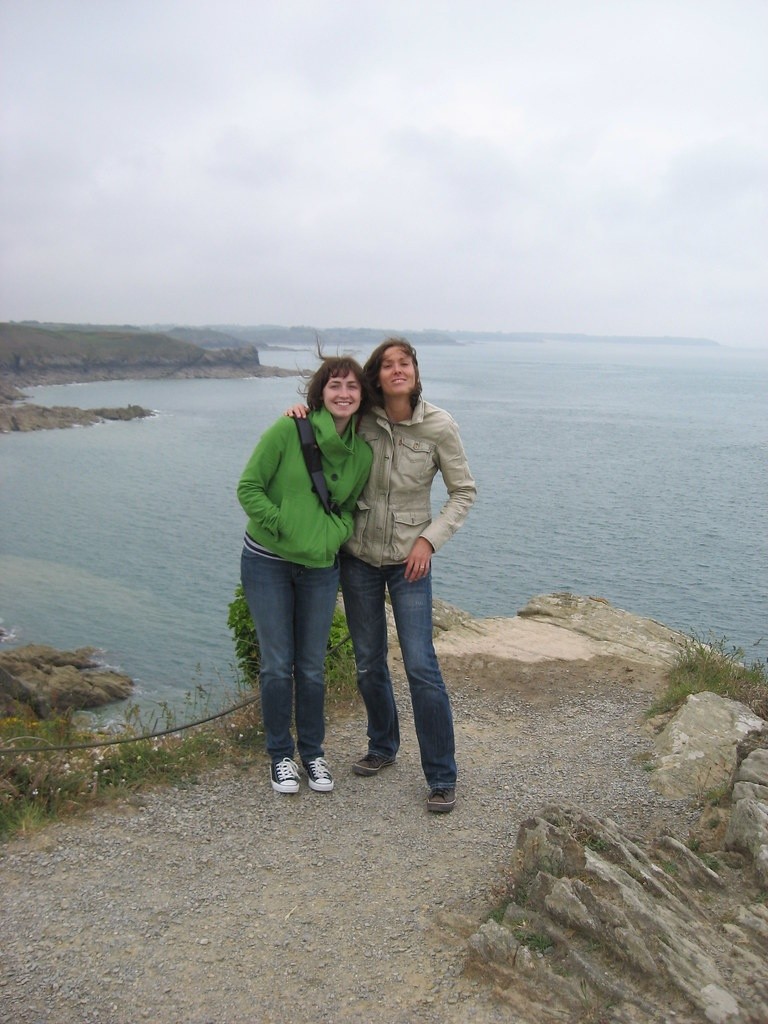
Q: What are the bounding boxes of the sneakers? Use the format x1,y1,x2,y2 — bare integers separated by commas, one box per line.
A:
426,787,456,812
355,754,397,776
300,759,336,792
270,761,300,793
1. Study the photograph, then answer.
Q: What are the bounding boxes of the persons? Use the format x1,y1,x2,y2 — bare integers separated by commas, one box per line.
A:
281,333,478,816
235,328,374,796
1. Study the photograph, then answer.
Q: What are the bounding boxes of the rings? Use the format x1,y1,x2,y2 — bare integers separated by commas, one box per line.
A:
420,565,425,569
